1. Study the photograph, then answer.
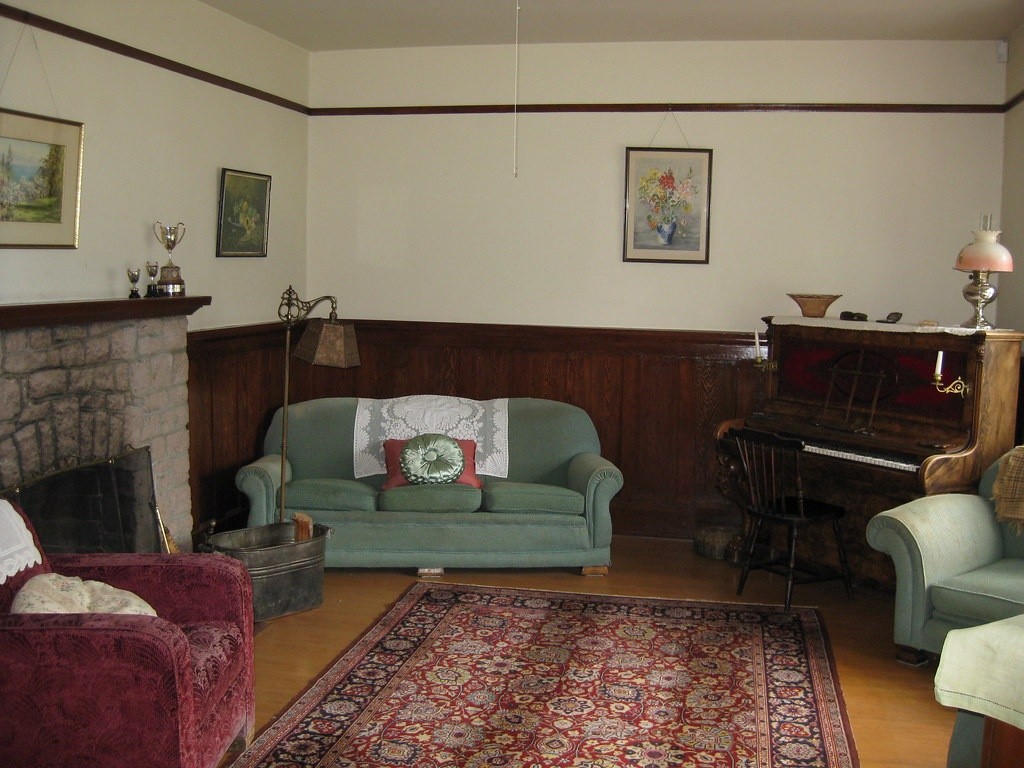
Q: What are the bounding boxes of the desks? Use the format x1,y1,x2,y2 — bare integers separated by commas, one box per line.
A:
934,613,1024,768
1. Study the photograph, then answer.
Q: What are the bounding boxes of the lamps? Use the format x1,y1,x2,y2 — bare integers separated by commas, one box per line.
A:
278,285,362,521
954,212,1013,330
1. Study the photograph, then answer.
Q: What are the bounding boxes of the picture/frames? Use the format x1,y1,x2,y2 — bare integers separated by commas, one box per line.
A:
623,147,713,264
215,168,272,257
0,107,86,249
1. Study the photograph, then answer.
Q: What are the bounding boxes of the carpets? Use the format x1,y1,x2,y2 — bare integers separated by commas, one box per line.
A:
218,580,861,768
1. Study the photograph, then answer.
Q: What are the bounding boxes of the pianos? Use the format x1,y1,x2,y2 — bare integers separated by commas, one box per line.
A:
715,314,1024,596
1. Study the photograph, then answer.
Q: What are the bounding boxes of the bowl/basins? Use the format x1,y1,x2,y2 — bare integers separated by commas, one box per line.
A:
787,293,843,317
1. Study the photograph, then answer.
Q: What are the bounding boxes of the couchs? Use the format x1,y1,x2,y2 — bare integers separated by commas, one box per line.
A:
0,498,256,768
866,446,1024,666
236,394,623,577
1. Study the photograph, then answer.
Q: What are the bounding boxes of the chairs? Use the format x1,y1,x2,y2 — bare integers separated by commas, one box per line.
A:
727,427,854,616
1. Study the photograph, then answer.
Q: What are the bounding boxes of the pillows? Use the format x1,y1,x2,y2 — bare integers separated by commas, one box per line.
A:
382,434,483,491
10,573,158,617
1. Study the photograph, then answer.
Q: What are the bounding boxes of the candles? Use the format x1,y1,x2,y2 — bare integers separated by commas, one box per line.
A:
754,328,761,357
935,351,944,376
